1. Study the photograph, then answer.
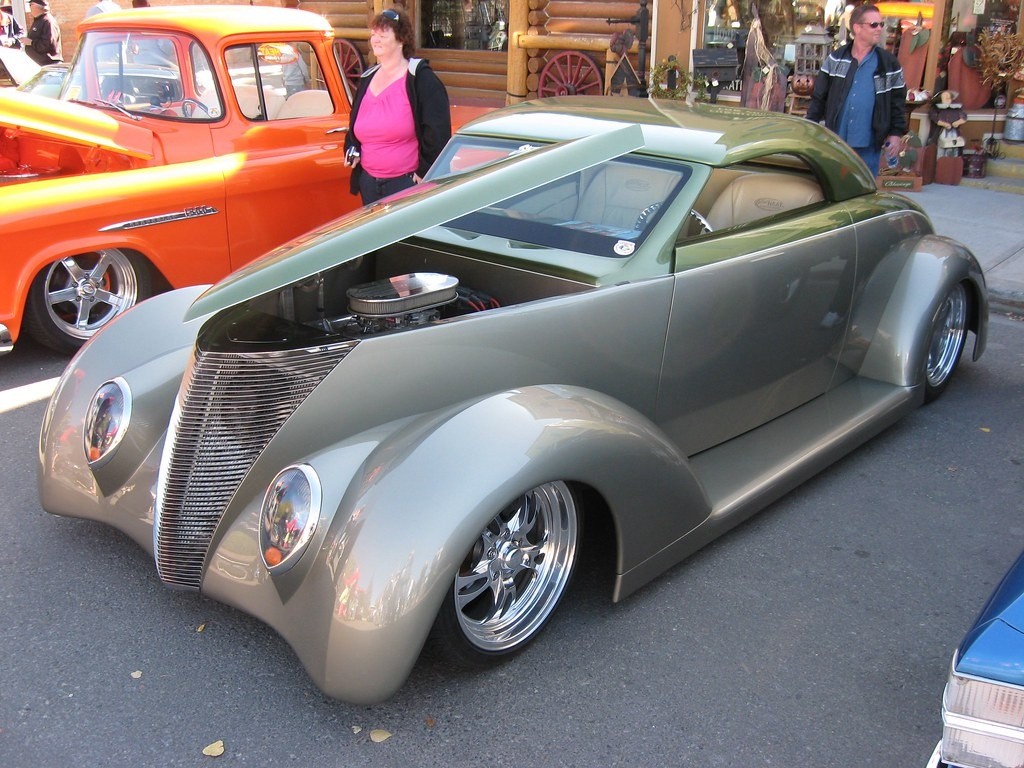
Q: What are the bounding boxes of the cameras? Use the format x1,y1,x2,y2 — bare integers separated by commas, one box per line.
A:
347,146,360,166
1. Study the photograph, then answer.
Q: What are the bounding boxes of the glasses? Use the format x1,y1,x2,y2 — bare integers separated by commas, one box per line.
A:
383,9,399,21
857,21,884,28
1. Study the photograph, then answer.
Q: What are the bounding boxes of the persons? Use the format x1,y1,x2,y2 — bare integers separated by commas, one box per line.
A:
86,0,123,60
343,8,451,207
126,0,178,69
930,91,968,156
805,5,907,177
20,0,63,67
0,11,25,48
280,42,310,98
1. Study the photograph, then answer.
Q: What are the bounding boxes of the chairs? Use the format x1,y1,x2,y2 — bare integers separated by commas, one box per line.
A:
699,171,826,233
186,84,276,118
574,165,685,231
277,89,334,119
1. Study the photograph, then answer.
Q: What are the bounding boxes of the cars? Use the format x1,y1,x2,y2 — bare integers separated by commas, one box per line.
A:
926,549,1024,768
1,5,516,360
8,62,202,112
36,94,990,707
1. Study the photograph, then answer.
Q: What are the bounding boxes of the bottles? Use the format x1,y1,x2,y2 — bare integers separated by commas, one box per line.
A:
884,142,898,168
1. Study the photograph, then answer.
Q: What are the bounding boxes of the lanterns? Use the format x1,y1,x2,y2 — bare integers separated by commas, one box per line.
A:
791,74,816,96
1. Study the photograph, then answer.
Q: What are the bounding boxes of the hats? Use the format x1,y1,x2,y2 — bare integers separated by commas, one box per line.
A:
29,0,47,6
